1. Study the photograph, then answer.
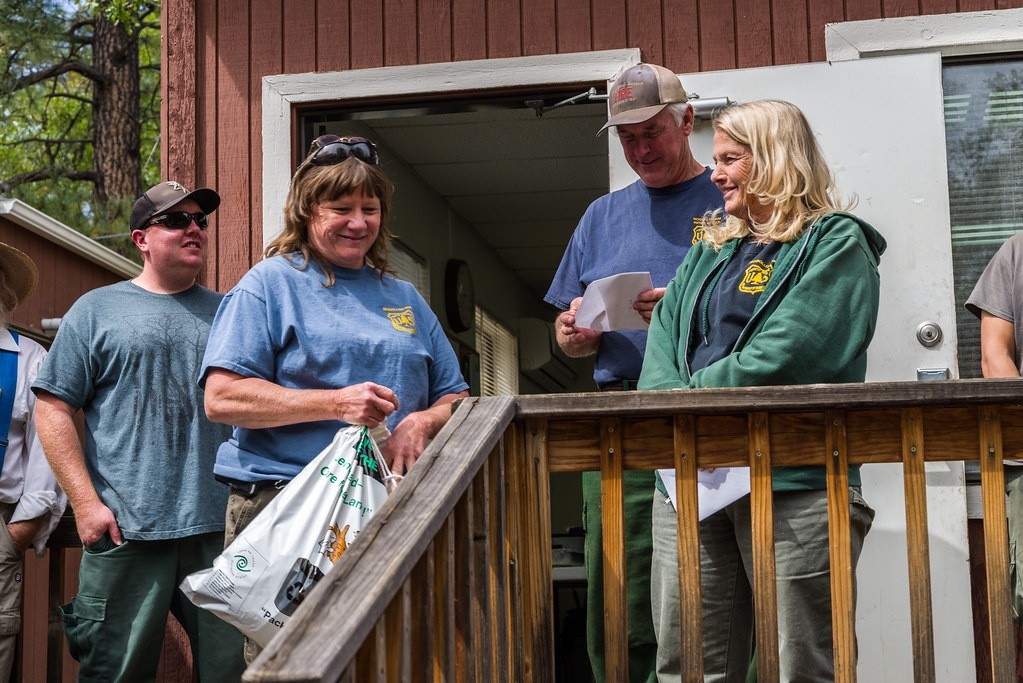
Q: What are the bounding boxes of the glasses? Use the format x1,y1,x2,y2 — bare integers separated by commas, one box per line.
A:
299,141,379,181
139,211,208,230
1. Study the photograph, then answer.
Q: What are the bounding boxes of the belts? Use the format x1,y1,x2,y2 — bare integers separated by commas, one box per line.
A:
600,380,637,391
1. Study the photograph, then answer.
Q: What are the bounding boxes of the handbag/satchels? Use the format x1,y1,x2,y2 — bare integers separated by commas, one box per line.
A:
179,412,406,649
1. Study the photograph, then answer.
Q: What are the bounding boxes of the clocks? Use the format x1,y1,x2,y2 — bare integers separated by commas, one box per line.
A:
443,258,475,334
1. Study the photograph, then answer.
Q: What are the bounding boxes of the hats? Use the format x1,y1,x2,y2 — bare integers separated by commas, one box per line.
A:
596,63,700,139
130,181,221,232
0,243,40,315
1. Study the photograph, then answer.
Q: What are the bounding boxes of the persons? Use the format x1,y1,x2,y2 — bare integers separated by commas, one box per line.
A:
545,64,759,683
637,100,887,683
963,231,1023,683
197,134,471,683
31,181,247,683
0,241,68,683
